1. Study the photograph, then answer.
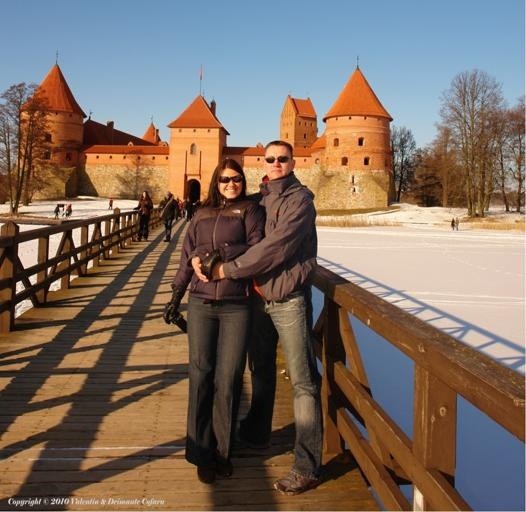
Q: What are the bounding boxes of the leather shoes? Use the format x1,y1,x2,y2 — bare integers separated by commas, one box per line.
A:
164,302,187,333
202,250,222,280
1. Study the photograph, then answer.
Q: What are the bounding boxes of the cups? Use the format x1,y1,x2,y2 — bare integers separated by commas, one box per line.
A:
265,156,288,163
219,176,242,182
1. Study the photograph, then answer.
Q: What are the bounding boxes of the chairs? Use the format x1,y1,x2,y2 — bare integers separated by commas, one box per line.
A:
275,473,319,496
235,430,271,452
164,235,171,242
136,236,147,241
197,457,234,484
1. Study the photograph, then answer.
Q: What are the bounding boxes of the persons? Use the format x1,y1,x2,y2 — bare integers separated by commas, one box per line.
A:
132,189,153,241
450,217,460,231
108,198,113,210
159,139,324,497
53,203,72,221
